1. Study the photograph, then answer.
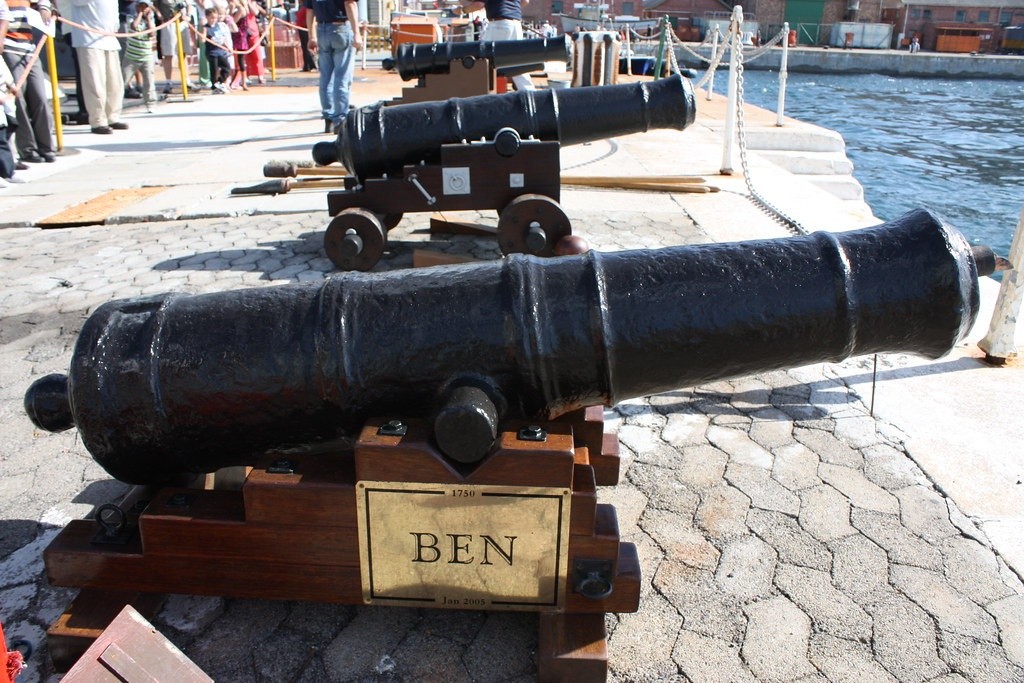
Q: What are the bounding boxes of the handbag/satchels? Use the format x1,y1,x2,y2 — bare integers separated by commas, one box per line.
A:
259,31,267,46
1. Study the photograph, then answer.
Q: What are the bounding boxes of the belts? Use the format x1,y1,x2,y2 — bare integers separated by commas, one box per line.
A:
489,17,513,21
318,19,347,23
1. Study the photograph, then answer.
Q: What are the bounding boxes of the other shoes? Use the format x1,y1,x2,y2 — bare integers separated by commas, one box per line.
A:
187,83,201,92
334,126,339,134
25,155,45,162
124,87,144,98
325,119,334,133
215,82,230,94
14,161,28,169
163,84,171,93
41,152,56,162
136,85,144,93
9,174,28,182
0,177,8,188
91,127,112,134
110,122,128,129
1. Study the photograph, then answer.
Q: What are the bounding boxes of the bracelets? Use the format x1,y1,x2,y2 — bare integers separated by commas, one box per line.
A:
461,8,466,14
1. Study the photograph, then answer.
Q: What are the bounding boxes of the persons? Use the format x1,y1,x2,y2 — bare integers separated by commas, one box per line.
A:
295,0,317,72
118,0,270,98
153,0,201,94
619,23,637,42
452,0,535,92
756,30,761,47
647,24,652,42
71,0,129,134
472,16,488,42
121,0,158,112
527,18,557,38
0,0,89,188
303,0,364,133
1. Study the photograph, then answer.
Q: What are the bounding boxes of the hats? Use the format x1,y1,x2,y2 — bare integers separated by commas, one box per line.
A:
138,0,149,7
